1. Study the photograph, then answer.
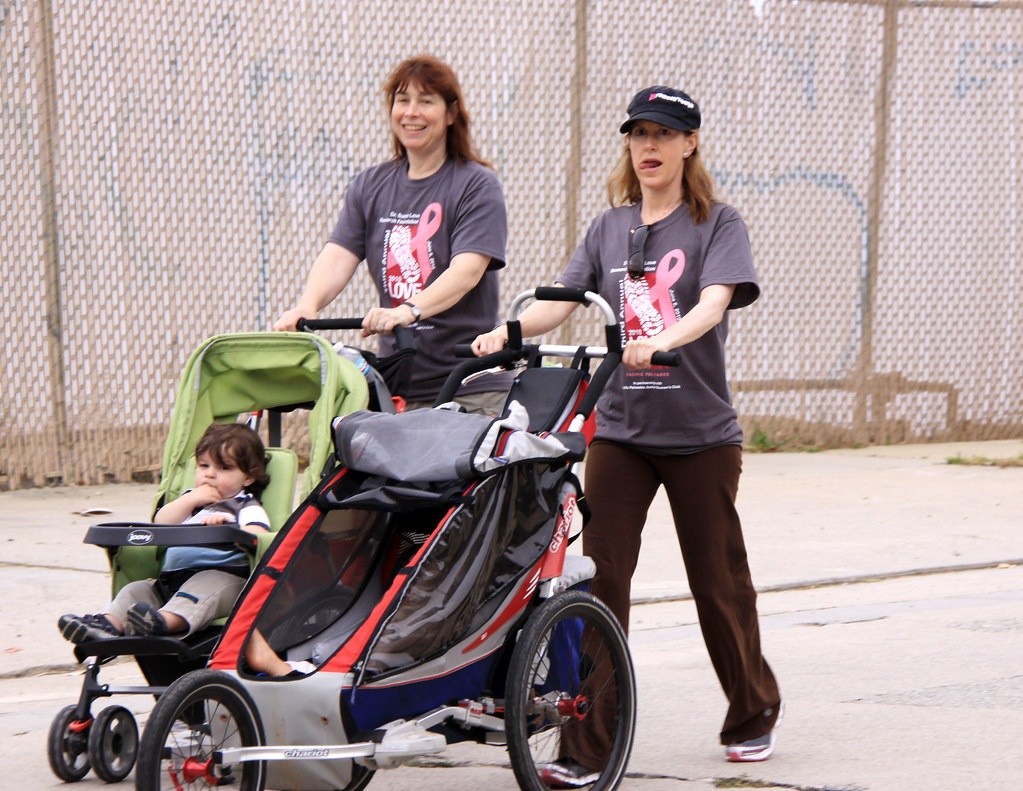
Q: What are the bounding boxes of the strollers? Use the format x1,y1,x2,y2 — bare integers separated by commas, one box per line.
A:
134,286,683,791
47,318,422,784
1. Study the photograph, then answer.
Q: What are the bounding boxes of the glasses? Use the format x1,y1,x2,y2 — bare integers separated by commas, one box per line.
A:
627,225,651,279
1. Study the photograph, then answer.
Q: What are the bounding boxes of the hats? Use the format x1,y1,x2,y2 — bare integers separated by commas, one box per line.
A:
619,85,701,133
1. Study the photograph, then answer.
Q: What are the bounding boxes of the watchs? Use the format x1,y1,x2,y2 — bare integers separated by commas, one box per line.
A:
402,301,421,325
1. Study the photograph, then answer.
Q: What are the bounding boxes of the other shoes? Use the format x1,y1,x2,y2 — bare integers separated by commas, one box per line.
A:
57,612,120,641
123,603,165,638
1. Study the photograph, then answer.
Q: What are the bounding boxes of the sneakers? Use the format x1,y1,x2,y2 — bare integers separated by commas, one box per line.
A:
725,698,784,761
536,757,602,786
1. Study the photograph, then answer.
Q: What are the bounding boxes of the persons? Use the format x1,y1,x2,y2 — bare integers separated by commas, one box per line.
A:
469,86,785,786
57,422,271,645
272,57,520,414
245,627,317,677
